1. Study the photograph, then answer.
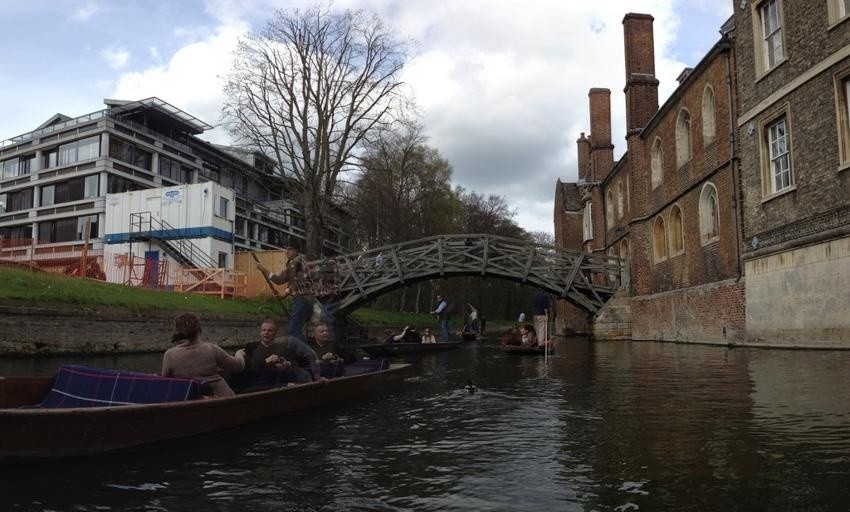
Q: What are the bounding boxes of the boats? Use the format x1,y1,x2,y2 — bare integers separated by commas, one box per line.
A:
462,331,489,343
360,342,460,359
0,363,416,458
500,339,557,353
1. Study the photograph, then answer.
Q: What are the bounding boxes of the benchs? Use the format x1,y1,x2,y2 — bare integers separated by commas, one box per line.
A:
19,364,201,412
307,358,390,380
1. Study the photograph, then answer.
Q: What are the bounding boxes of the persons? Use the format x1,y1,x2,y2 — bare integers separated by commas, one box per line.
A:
159,311,246,396
422,327,436,344
531,288,548,348
430,293,451,342
309,321,345,380
255,237,316,366
383,325,409,344
510,310,537,346
401,325,422,343
463,301,486,336
253,318,312,390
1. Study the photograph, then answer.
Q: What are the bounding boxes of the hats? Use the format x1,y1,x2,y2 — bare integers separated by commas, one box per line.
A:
175,313,200,333
